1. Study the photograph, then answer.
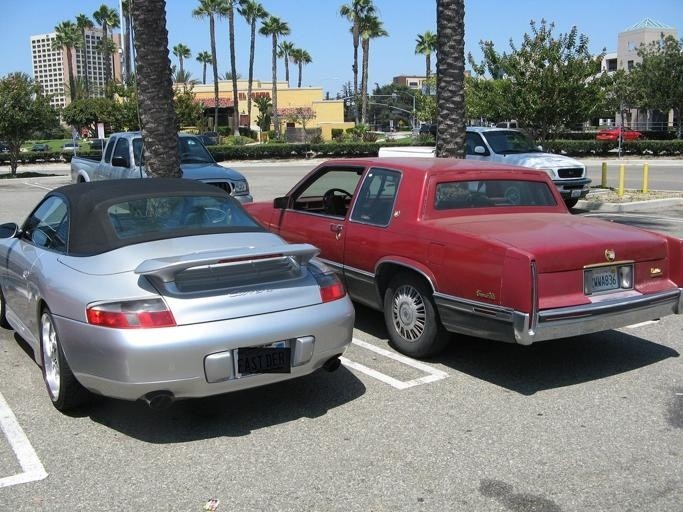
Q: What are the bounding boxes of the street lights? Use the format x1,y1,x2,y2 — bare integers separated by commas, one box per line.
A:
342,95,356,106
392,91,416,128
117,46,124,103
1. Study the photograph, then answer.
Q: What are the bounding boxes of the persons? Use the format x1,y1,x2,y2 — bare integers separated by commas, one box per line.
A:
82,126,89,139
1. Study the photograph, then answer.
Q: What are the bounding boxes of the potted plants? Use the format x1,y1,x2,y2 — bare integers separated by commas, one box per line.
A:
253,114,270,142
